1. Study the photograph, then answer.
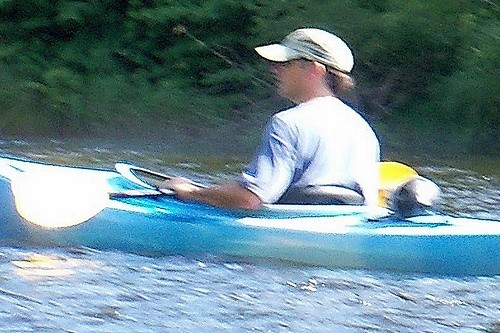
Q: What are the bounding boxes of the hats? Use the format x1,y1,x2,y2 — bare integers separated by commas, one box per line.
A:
254,28,355,72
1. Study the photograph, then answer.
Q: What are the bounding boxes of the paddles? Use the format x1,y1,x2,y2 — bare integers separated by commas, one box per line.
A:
10,168,174,227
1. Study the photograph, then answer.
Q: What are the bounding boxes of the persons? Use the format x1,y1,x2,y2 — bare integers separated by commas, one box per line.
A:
159,28,380,214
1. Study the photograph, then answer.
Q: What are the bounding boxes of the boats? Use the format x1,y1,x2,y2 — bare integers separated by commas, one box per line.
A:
0,152,500,280
